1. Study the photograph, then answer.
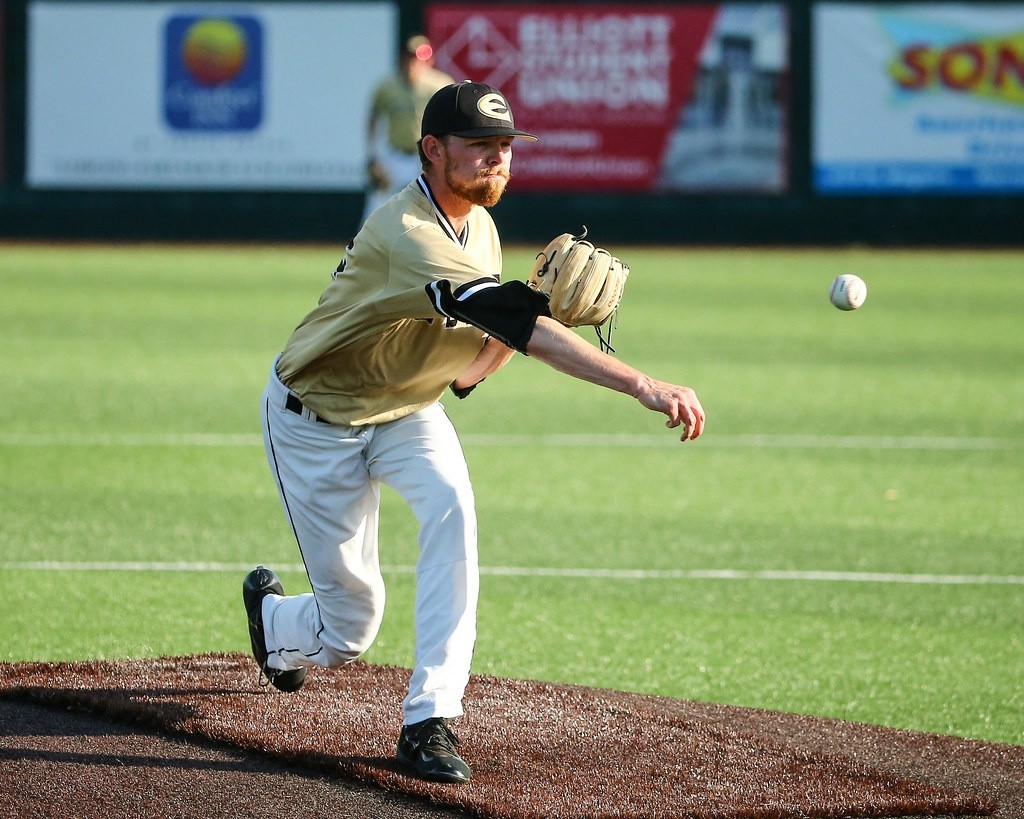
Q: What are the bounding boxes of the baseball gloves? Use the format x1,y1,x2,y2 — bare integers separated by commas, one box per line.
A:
529,233,631,328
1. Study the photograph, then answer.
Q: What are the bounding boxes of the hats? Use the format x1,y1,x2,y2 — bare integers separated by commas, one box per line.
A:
405,36,434,58
421,78,539,143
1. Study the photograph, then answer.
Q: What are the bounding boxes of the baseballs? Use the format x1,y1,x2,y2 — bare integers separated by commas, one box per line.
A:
829,274,868,312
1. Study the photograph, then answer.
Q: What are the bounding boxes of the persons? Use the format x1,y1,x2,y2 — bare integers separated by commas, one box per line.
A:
243,82,706,780
362,36,454,220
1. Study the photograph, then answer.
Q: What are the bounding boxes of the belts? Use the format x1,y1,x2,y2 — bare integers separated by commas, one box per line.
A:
287,394,334,425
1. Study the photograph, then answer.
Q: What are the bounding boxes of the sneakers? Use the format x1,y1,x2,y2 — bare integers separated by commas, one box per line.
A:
397,714,470,781
244,567,307,694
